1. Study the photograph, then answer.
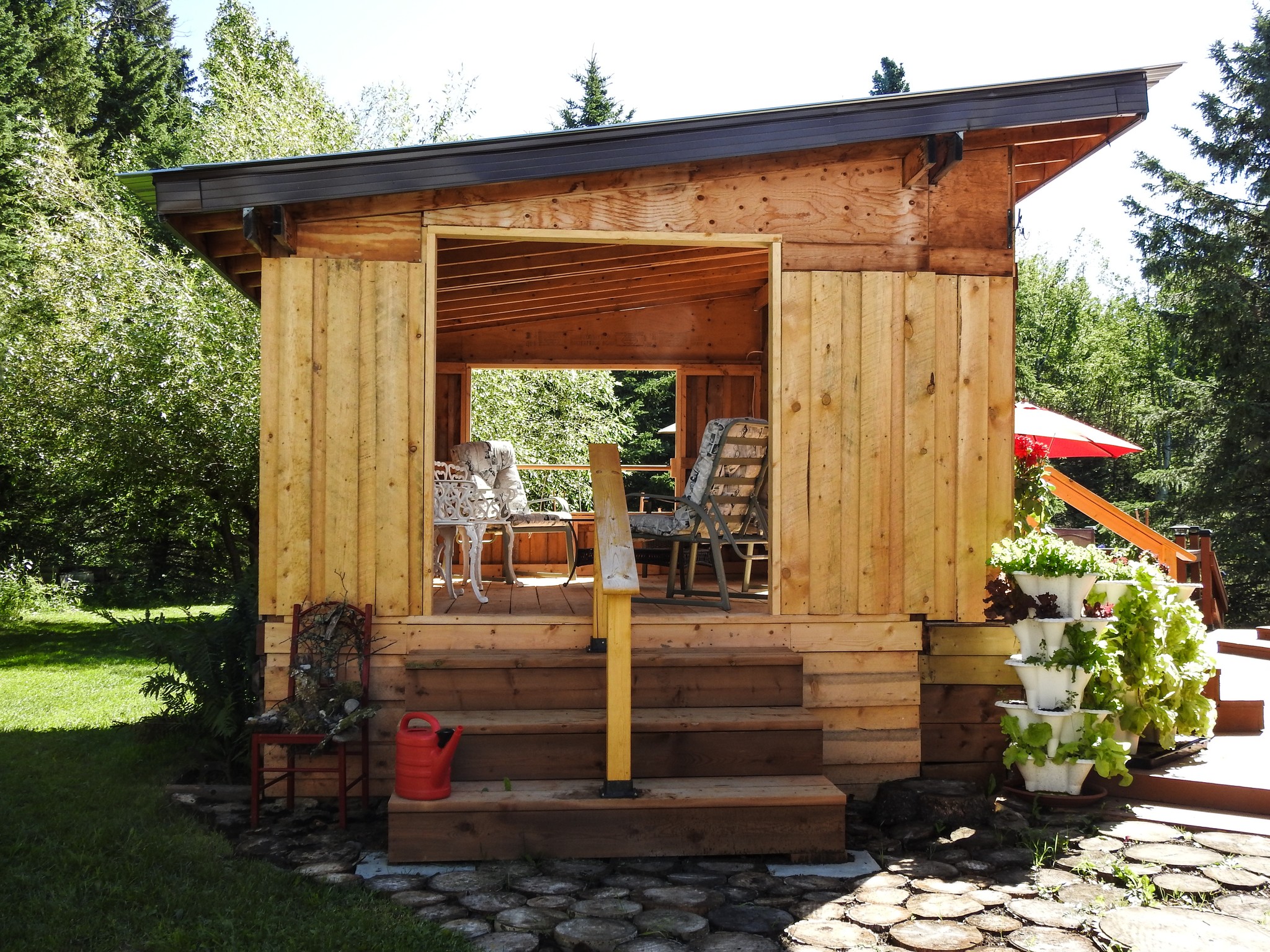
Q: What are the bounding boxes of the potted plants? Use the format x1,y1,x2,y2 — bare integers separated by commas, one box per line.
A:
988,525,1218,794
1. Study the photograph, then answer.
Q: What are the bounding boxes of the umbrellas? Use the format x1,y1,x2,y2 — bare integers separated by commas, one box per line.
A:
1015,395,1145,486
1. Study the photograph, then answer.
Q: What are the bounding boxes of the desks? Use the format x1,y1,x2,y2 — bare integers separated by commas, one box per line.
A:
560,519,595,586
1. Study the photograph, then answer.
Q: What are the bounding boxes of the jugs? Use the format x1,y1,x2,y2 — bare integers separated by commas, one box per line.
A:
395,711,464,801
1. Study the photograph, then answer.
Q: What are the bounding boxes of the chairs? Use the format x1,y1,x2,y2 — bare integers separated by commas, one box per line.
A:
434,461,523,602
249,603,373,828
631,417,768,610
454,440,580,580
